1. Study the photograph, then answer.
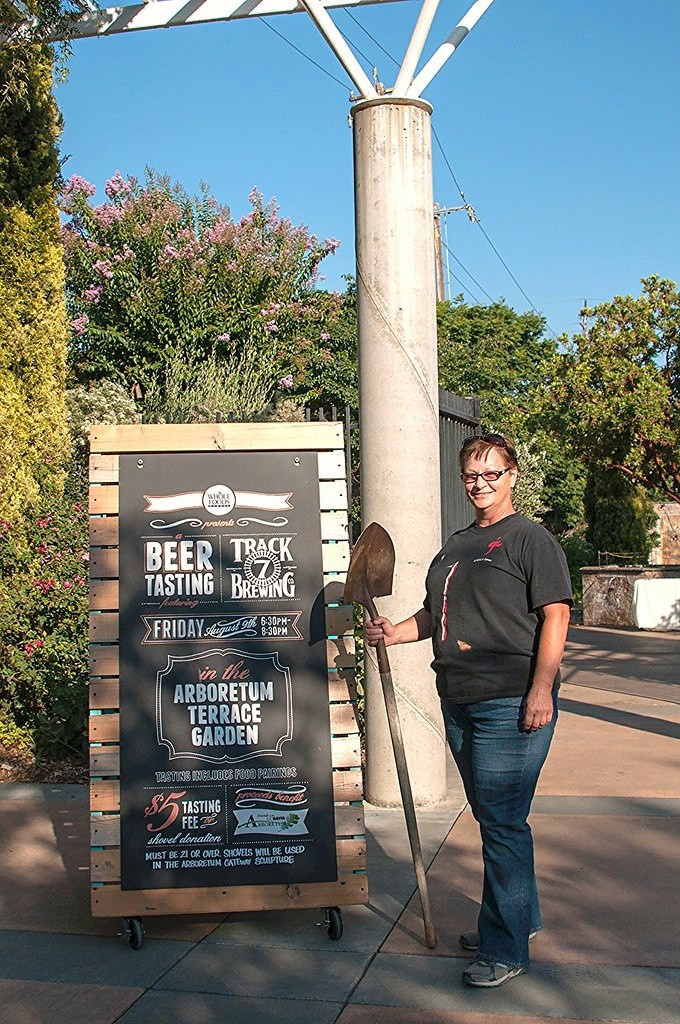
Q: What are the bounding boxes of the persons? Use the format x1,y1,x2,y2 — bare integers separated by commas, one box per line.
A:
363,435,573,986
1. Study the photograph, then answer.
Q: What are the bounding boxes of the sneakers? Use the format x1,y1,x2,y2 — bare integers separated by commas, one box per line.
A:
459,929,537,951
463,954,531,986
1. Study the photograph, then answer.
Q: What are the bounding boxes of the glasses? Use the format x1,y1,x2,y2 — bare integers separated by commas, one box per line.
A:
462,434,513,456
460,468,509,484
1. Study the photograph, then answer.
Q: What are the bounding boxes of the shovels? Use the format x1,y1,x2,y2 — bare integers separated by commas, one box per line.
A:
342,520,438,948
